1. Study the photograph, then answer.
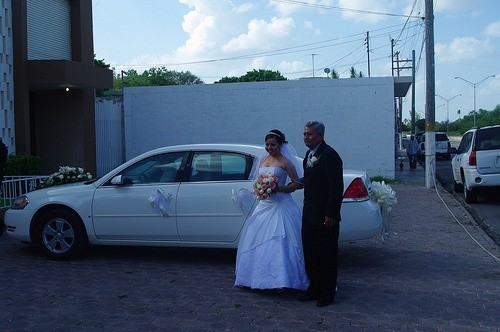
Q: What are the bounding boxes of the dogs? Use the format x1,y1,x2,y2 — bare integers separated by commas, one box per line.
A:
399,160,404,171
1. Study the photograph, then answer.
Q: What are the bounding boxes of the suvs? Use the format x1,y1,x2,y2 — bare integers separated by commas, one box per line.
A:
402,130,452,161
450,124,500,204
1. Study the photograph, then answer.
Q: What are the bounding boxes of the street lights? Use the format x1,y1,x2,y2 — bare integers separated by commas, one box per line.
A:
454,75,496,127
434,94,463,134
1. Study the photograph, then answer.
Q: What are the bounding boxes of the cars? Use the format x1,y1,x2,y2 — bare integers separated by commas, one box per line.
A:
3,142,386,261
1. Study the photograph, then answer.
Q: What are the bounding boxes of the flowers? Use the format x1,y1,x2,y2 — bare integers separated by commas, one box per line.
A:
32,164,93,190
251,173,279,200
310,156,318,163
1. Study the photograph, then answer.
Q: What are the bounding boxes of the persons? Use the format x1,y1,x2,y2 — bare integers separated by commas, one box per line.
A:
234,129,311,290
288,120,344,307
407,135,419,170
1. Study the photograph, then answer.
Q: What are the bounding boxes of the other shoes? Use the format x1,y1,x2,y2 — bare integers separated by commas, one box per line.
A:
299,295,314,301
316,300,332,306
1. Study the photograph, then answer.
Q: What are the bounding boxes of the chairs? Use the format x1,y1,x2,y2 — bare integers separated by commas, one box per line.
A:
158,159,178,181
192,159,211,181
